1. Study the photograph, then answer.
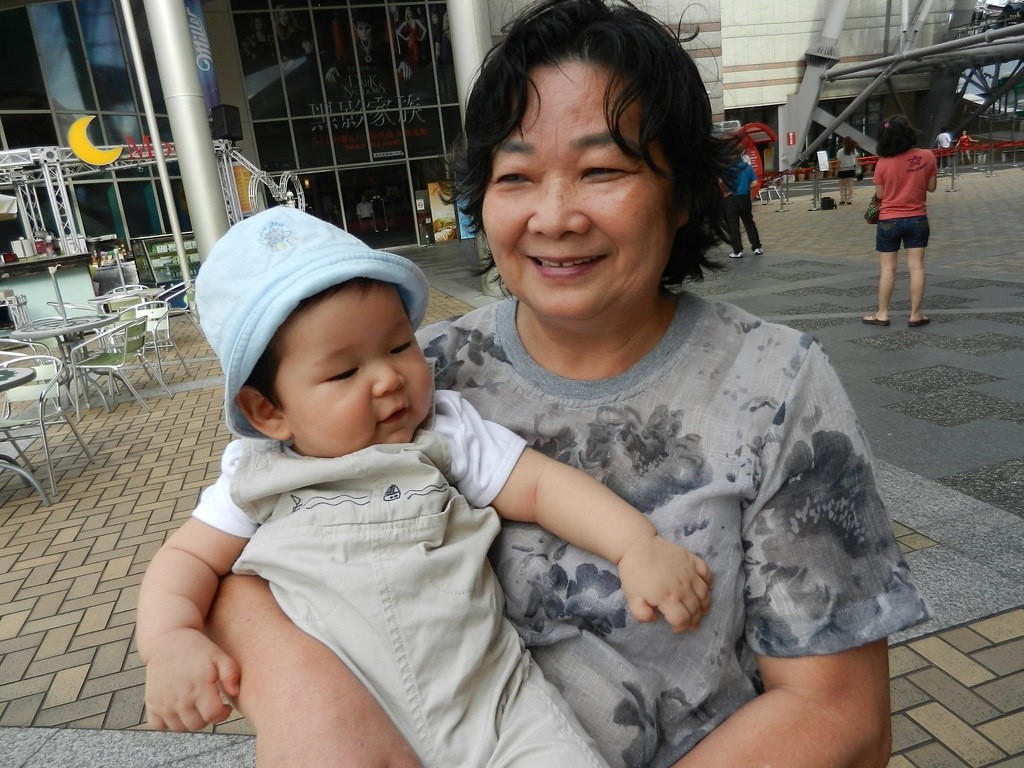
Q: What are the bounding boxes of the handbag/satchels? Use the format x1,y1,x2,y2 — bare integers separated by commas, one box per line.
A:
855,166,862,177
864,192,881,224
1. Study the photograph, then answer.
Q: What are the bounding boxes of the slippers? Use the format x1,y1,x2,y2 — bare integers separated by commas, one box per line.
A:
862,314,890,326
908,315,930,327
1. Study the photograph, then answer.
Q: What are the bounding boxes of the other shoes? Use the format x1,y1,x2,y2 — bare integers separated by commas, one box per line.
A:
846,202,851,205
839,201,845,205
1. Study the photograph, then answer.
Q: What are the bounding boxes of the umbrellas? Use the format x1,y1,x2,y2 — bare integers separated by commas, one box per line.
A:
0,195,18,221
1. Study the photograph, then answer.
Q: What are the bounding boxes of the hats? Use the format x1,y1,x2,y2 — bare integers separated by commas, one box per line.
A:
195,205,430,440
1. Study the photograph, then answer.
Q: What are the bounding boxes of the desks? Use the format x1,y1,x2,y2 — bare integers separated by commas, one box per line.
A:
89,288,165,353
0,367,37,393
10,312,119,411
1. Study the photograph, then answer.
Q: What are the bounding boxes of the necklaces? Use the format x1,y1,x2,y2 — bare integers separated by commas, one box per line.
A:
362,40,372,63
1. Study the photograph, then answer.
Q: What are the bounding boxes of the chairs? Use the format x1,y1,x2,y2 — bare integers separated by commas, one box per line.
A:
0,277,205,509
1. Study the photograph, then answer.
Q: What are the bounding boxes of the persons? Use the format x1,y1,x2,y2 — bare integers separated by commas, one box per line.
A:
717,151,764,257
935,127,952,167
357,195,376,246
955,130,980,165
203,0,931,768
135,206,715,768
242,0,461,134
835,136,858,205
862,116,937,327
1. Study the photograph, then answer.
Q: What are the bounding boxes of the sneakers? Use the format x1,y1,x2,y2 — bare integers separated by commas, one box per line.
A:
729,251,743,258
754,248,764,255
